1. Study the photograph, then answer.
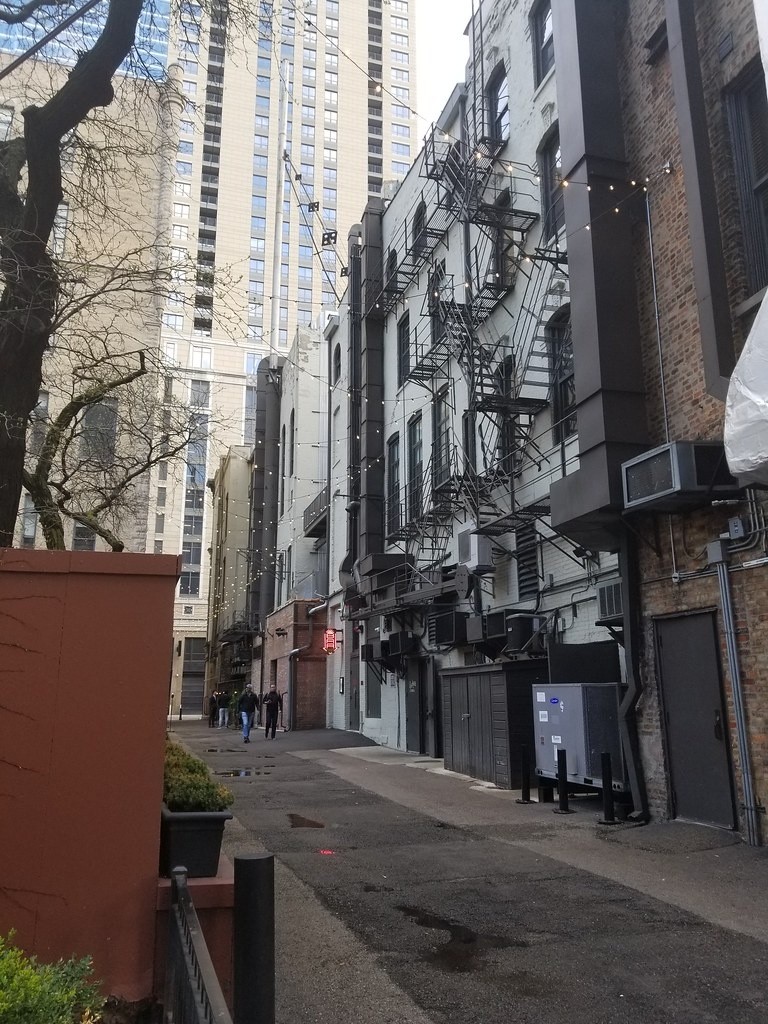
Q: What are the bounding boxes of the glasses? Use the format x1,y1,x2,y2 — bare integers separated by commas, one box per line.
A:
246,687,250,689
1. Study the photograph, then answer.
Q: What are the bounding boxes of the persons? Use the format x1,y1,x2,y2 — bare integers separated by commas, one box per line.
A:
218,691,230,729
209,690,218,727
236,684,260,743
262,684,283,740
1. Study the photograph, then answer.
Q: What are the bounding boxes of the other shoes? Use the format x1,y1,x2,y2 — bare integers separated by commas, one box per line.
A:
270,736,276,741
209,726,212,728
212,725,216,727
224,726,228,728
217,726,222,730
244,738,248,743
265,737,268,740
247,738,250,743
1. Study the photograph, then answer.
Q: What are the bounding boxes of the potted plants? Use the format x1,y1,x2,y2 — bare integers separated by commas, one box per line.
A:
159,741,233,878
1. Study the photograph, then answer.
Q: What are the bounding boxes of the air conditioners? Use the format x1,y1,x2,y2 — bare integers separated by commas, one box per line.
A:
389,630,413,654
457,518,493,567
596,579,625,619
434,611,470,646
623,437,736,506
